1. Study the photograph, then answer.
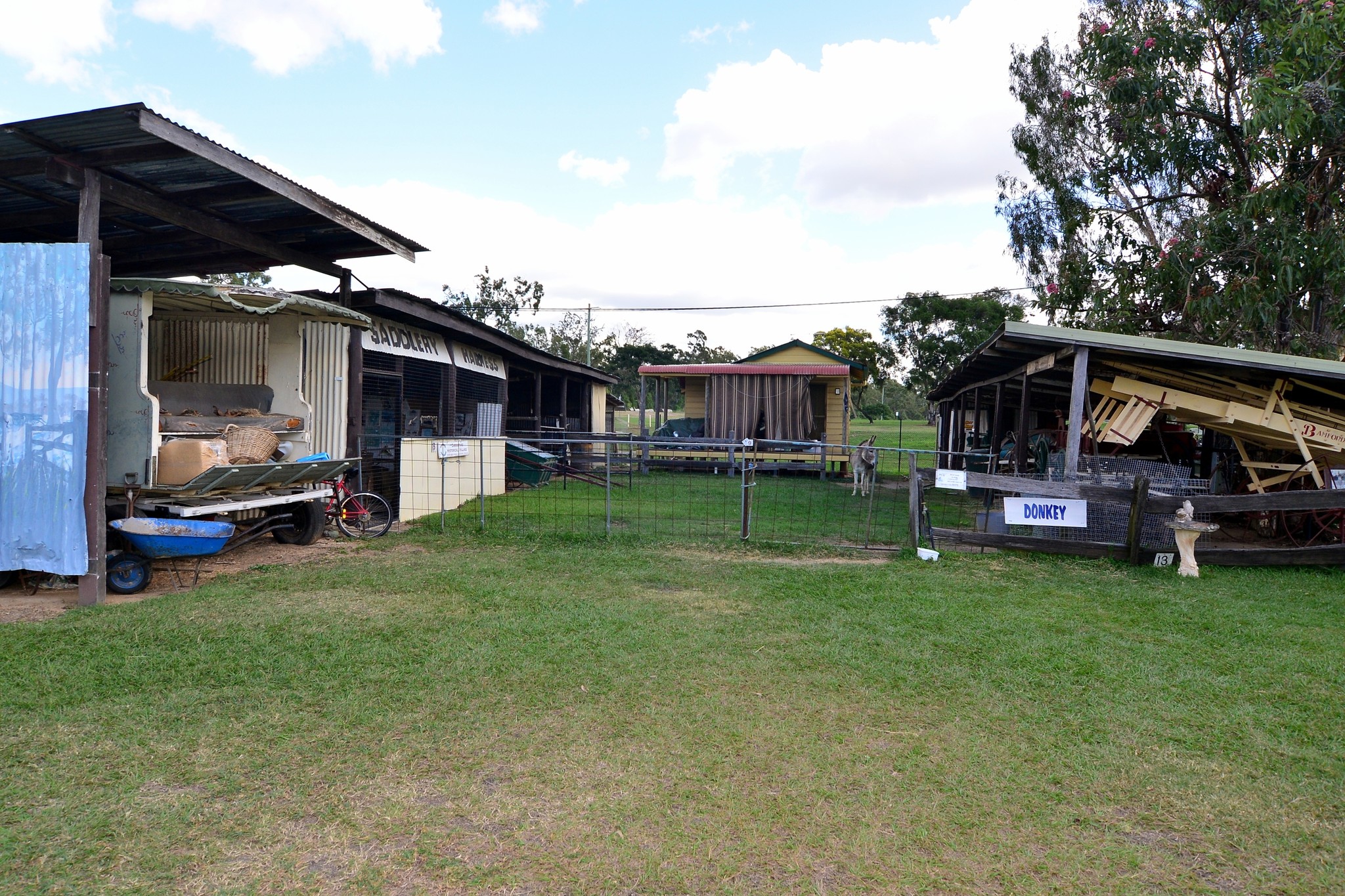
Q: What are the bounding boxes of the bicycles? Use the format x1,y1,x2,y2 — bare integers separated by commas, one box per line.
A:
318,467,393,540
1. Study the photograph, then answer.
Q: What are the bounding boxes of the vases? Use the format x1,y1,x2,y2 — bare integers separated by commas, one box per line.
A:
976,512,1010,534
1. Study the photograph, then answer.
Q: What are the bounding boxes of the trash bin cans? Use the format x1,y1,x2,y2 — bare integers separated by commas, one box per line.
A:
974,512,1010,536
961,449,989,501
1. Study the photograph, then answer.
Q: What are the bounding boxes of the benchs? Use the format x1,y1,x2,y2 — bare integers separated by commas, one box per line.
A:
146,380,304,434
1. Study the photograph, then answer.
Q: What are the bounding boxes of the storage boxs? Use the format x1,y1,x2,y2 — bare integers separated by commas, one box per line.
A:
505,437,558,487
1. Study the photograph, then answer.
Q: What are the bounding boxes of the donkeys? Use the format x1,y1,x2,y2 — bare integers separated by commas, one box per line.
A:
850,436,878,496
